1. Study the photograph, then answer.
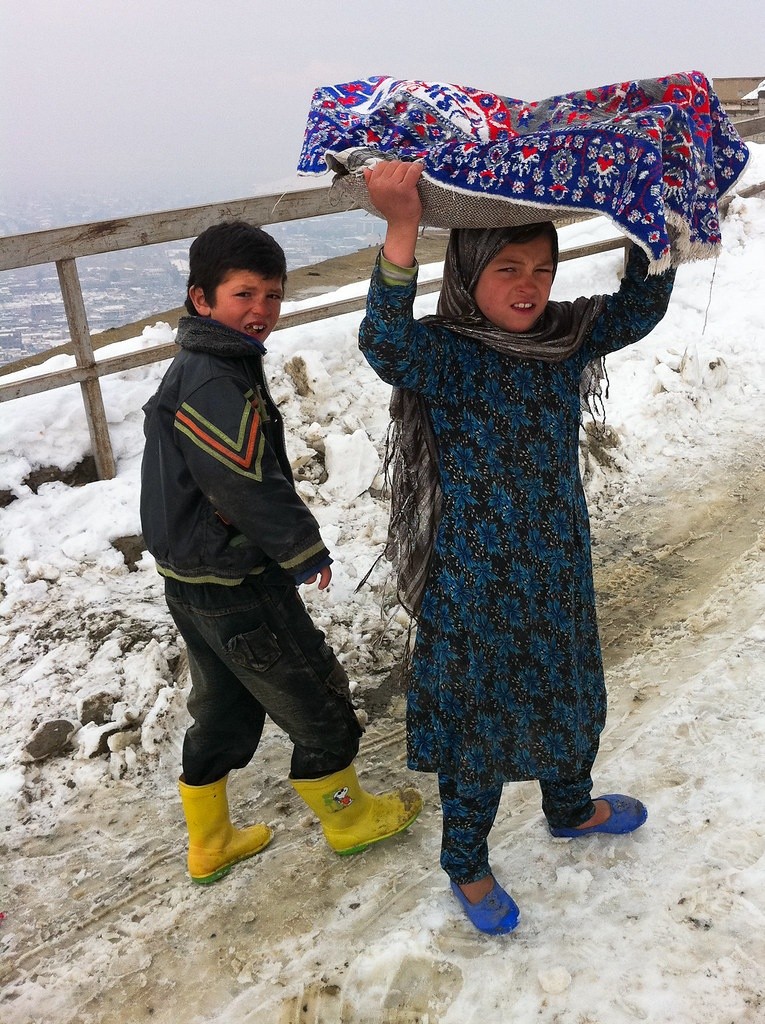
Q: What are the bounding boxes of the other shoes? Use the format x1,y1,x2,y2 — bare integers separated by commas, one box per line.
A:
550,794,648,837
450,877,520,935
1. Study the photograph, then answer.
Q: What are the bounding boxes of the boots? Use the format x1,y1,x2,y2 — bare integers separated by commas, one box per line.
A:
287,763,423,856
179,773,275,884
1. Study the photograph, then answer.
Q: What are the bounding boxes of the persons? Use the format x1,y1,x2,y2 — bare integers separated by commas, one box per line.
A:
358,160,679,934
141,221,424,883
